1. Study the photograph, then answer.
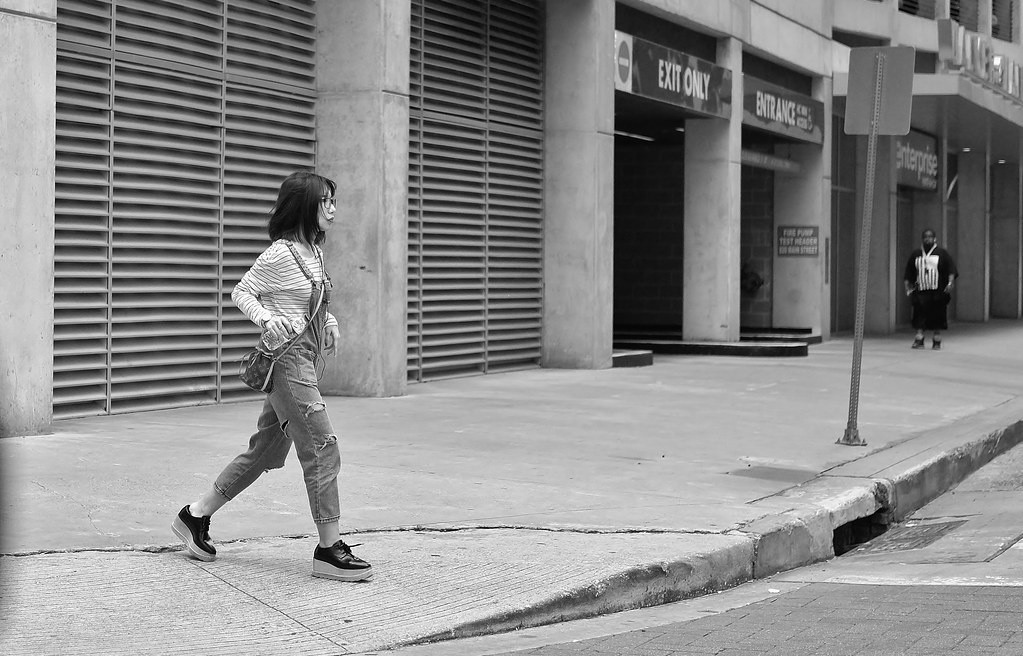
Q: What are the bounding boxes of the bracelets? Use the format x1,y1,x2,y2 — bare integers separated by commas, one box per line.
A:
948,282,953,286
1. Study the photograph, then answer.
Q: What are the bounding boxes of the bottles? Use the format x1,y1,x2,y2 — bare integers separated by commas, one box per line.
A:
262,315,310,351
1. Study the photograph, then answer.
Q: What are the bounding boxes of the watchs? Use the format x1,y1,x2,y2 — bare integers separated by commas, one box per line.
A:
261,312,274,327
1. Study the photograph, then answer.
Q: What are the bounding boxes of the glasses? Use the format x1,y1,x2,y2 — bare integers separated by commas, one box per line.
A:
320,196,338,209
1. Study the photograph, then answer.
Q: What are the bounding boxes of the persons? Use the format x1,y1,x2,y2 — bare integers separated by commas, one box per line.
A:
173,171,373,580
904,229,959,350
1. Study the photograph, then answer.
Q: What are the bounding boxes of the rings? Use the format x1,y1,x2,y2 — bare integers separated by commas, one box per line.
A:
279,326,283,329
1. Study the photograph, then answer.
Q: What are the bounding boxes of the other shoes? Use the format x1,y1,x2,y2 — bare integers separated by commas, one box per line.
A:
932,335,943,350
912,335,925,349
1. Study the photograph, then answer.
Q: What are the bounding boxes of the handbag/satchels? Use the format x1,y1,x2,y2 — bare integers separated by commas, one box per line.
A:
238,346,278,393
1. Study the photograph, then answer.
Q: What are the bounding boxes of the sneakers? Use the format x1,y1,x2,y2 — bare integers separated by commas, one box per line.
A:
171,505,216,562
311,540,373,582
